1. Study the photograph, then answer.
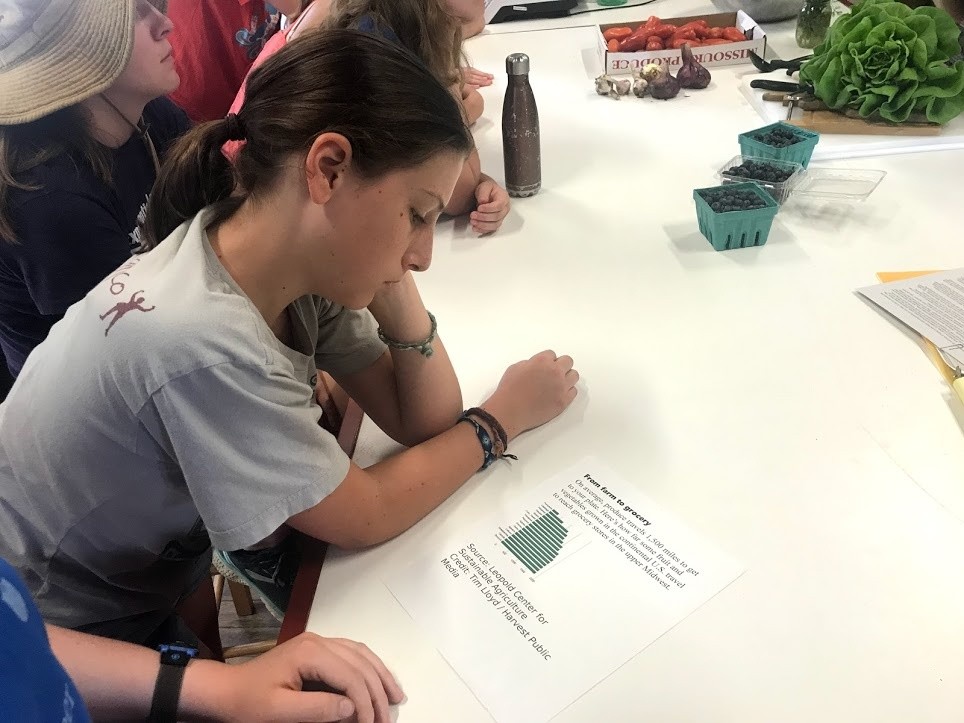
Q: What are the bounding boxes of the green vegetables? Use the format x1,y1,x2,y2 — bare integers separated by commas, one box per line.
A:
799,0,964,123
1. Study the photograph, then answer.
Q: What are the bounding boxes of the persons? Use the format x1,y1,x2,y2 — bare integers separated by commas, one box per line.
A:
0,30,580,635
0,0,302,624
165,0,510,235
0,558,403,723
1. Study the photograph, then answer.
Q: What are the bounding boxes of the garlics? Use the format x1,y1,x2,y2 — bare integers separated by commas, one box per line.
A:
650,43,711,99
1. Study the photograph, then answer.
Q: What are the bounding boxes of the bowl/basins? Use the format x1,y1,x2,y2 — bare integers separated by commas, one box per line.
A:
710,0,804,21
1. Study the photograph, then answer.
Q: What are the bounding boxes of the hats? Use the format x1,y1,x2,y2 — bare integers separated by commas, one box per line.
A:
0,1,168,125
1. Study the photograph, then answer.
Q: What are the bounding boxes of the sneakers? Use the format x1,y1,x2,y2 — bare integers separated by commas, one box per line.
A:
212,537,298,622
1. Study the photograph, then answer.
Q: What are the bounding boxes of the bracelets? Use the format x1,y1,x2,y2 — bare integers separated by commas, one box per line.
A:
377,310,438,358
457,408,508,471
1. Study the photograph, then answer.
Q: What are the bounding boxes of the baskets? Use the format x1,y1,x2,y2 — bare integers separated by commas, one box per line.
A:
692,181,778,250
739,121,819,171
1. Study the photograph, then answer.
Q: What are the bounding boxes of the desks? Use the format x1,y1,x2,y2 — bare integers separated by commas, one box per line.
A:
276,0,964,723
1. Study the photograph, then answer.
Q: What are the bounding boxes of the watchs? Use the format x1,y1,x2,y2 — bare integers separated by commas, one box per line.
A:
149,641,200,723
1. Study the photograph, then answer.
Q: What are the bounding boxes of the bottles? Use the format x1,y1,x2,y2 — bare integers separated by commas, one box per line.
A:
795,0,833,49
502,53,541,198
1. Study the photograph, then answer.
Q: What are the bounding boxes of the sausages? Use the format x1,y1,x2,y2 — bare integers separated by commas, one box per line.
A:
603,16,748,52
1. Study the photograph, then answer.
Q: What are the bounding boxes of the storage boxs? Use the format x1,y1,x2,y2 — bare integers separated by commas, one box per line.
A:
694,181,781,250
591,8,767,74
738,120,820,172
